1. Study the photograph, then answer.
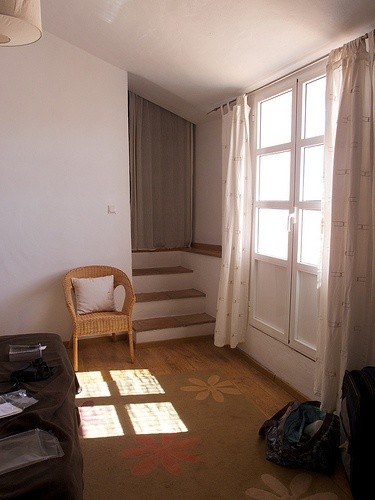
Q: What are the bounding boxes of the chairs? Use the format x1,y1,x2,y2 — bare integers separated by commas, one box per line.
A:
62,266,137,372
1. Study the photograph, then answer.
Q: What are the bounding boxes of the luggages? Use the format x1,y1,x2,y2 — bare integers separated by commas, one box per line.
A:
339,366,375,500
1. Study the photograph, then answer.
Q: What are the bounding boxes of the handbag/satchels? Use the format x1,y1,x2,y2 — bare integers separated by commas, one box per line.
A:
0,358,56,394
258,401,340,477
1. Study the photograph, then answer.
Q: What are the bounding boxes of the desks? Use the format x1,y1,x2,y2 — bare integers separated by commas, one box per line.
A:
0,333,84,500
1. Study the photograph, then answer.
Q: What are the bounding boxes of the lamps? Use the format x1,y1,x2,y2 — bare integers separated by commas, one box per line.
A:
0,0,43,48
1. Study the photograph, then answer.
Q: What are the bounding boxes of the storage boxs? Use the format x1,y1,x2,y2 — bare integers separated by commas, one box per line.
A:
340,366,375,500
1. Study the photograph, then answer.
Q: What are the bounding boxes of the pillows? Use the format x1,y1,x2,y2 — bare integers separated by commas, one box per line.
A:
70,274,115,316
